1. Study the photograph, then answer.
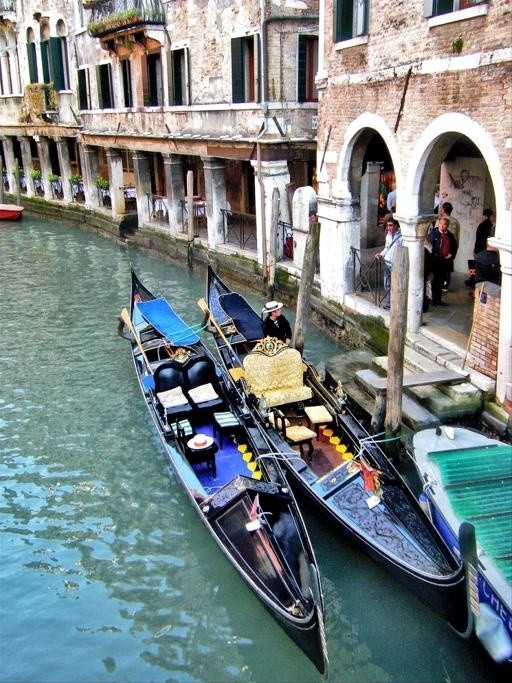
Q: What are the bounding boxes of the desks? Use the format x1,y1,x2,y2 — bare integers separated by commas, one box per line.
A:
2,170,231,231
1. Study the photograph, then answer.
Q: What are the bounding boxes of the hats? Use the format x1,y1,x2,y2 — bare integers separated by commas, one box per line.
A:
262,301,283,313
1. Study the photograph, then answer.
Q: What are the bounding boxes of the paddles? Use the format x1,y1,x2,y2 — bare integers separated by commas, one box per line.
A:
119,306,154,377
140,374,166,432
196,298,245,372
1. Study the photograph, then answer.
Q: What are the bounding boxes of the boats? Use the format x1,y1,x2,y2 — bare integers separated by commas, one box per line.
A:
117,266,328,681
409,423,512,667
0,203,25,220
201,257,479,639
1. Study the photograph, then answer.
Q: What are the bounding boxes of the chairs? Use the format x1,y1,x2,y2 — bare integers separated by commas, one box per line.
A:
185,356,225,415
273,408,318,463
174,418,217,478
152,362,193,419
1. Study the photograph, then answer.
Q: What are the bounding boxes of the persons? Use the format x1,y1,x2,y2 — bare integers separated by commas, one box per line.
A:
309,209,318,223
374,216,404,310
379,188,502,314
260,300,293,346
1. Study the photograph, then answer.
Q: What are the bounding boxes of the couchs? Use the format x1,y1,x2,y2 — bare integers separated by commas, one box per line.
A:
248,335,315,411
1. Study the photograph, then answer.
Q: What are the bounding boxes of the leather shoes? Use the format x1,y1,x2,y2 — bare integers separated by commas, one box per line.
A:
432,300,447,306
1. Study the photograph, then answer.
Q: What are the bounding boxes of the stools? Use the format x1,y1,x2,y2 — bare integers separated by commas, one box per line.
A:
212,411,238,450
266,410,289,429
170,419,196,436
304,405,335,441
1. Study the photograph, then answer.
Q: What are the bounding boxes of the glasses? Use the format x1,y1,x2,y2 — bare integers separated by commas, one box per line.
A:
386,224,394,226
